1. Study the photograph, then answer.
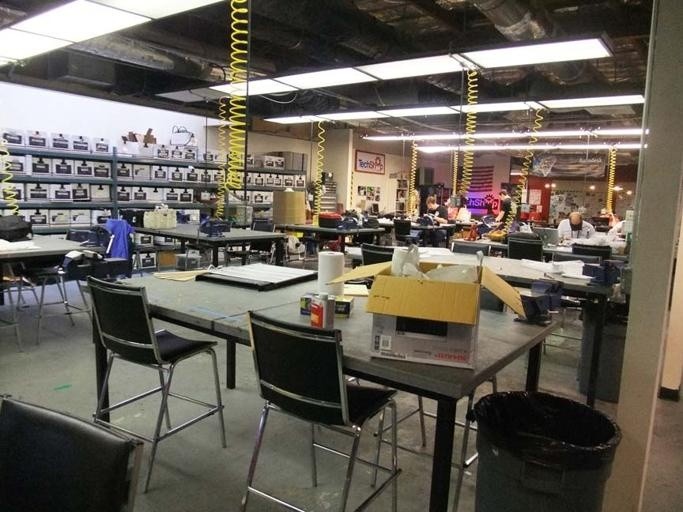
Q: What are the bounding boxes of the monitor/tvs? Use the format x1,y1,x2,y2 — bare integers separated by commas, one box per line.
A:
534,228,560,248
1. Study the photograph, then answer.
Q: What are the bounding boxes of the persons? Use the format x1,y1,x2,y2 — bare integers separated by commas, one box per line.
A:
494,190,519,229
415,194,449,246
556,211,596,240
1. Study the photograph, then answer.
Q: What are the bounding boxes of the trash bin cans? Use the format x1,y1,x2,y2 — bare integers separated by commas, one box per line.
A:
473,301,628,512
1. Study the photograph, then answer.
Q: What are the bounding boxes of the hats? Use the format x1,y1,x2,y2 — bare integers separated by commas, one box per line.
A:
569,212,581,225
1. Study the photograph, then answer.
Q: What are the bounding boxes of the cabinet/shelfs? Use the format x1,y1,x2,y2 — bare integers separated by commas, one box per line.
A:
419,184,453,216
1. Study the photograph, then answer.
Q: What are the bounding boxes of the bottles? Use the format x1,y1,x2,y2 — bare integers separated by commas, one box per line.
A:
310,293,335,329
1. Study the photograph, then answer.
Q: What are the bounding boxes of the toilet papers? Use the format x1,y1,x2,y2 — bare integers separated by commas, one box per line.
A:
390,247,408,276
318,251,345,297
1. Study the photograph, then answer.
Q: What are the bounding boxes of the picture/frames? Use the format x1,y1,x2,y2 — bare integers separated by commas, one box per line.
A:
355,150,385,174
